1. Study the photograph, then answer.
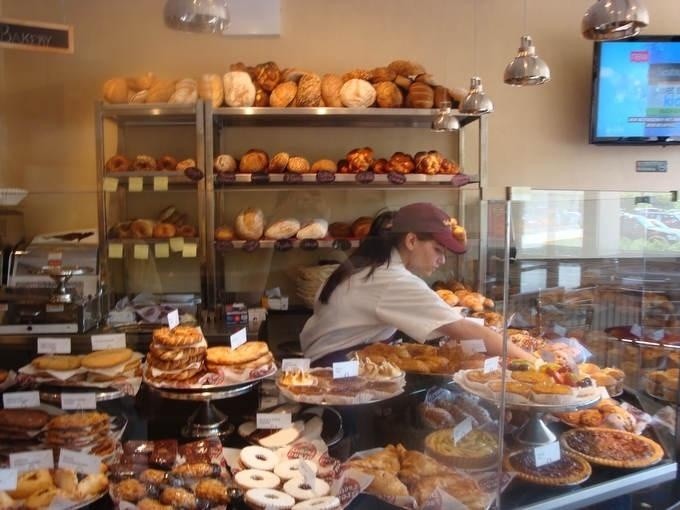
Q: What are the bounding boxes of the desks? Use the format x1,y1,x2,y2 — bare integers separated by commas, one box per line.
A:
2,344,680,509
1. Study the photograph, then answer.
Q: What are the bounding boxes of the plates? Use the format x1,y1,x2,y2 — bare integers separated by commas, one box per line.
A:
164,294,195,302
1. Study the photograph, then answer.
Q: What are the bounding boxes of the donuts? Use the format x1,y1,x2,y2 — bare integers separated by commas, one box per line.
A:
104,152,199,170
235,445,340,510
107,205,198,238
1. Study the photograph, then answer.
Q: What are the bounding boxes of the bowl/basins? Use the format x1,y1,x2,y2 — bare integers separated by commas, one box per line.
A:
294,265,341,310
0,188,29,208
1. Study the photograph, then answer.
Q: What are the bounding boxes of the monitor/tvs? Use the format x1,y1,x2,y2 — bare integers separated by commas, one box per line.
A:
589,35,680,145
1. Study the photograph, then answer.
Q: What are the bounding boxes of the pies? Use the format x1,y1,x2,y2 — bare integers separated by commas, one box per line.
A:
0,327,277,461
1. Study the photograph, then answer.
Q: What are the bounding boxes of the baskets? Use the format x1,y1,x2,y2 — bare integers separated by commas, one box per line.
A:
295,264,340,310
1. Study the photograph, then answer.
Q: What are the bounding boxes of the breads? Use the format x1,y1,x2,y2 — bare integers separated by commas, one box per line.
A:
0,463,228,510
560,427,663,470
576,363,625,396
464,352,596,405
553,400,637,432
352,341,490,375
431,282,494,312
215,148,460,174
103,60,452,106
507,329,580,353
214,208,375,241
648,367,680,401
341,443,489,510
418,396,531,431
425,428,500,468
504,446,591,486
279,356,402,403
470,312,505,327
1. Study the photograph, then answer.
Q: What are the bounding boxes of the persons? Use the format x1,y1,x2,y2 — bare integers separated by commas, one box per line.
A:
297,203,543,371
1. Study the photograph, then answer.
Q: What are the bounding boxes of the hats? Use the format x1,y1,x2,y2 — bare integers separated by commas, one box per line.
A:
391,203,472,253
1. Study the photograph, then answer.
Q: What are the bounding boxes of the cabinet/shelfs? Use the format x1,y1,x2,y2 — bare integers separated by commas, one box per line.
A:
93,96,207,331
2,241,119,353
201,100,491,324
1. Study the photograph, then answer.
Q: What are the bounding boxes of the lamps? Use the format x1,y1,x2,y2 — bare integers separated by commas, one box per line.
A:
456,0,498,122
573,2,648,45
161,0,236,38
425,0,462,137
499,0,550,91
